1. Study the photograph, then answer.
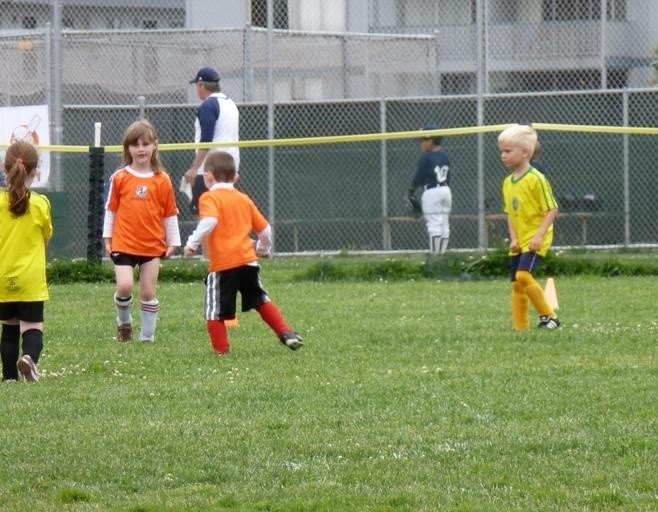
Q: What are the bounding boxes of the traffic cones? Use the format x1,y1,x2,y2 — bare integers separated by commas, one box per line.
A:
544,278,559,310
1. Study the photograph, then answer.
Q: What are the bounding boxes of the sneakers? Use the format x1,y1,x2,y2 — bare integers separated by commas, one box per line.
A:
115,323,133,344
280,332,305,350
537,315,561,330
16,355,40,384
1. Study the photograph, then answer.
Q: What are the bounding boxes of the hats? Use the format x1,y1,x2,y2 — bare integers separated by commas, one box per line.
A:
189,67,220,84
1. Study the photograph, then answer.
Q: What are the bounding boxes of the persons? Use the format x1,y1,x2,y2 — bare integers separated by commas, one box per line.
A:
102,119,182,343
183,151,304,355
497,125,561,331
184,67,240,225
408,125,452,255
0,141,53,384
